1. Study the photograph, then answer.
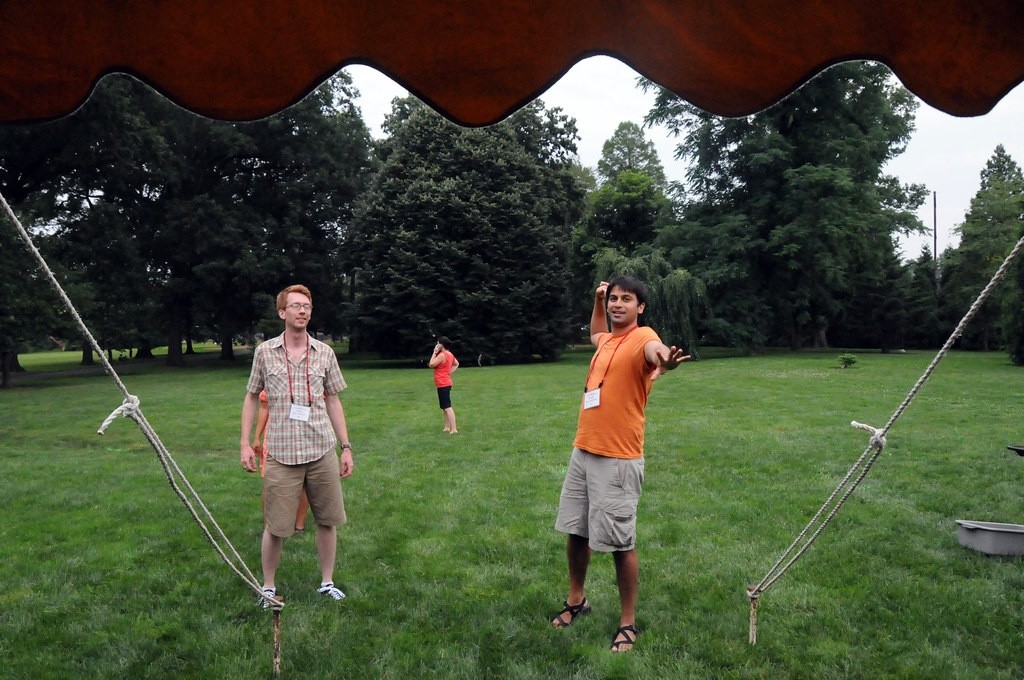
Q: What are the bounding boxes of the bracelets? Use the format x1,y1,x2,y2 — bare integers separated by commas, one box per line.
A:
433,352,436,354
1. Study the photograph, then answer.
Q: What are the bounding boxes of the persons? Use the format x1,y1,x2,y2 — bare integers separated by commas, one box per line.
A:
551,276,691,653
240,285,354,611
429,336,459,435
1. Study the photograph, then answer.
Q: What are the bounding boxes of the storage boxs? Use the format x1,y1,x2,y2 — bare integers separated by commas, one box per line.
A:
954,519,1024,555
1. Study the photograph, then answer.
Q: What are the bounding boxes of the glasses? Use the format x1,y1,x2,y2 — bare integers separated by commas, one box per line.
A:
283,303,311,310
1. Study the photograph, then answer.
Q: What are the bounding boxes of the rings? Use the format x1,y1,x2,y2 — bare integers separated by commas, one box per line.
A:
244,466,246,469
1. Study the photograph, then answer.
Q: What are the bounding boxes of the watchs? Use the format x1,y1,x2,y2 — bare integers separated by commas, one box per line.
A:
341,443,352,451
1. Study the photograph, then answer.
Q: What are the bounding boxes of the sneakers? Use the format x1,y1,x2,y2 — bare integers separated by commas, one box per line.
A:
317,582,346,600
256,587,277,608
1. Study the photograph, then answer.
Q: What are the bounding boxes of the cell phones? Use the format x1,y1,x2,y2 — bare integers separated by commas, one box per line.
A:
437,344,443,349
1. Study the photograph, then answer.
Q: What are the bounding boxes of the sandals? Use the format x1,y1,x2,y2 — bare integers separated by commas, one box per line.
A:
611,625,639,652
552,597,591,628
